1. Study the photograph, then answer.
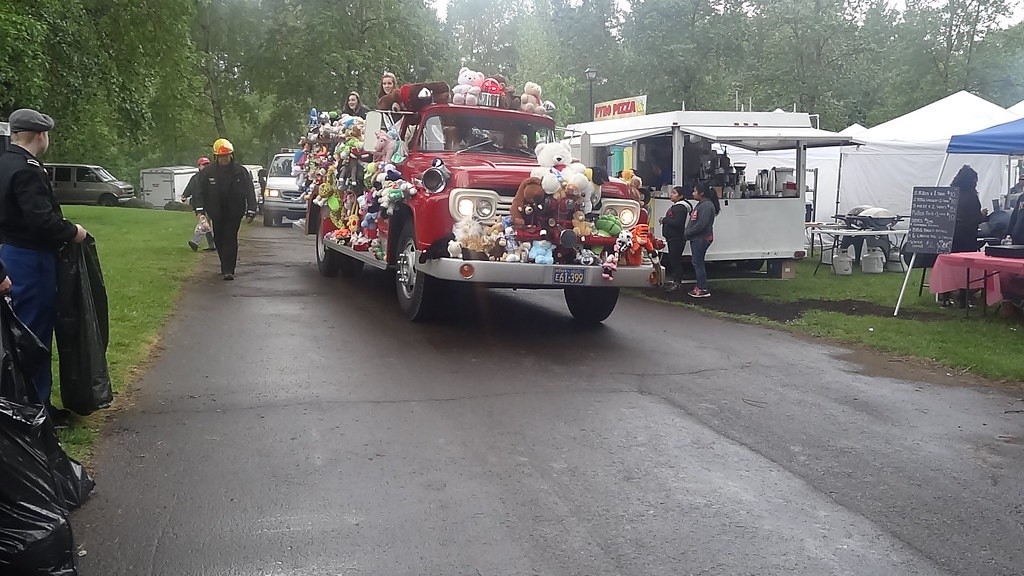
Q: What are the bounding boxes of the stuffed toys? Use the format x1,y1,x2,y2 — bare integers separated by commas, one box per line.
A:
377,67,555,125
419,141,665,286
291,108,417,261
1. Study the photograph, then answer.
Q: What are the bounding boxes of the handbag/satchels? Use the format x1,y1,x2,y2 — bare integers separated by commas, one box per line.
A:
195,215,213,235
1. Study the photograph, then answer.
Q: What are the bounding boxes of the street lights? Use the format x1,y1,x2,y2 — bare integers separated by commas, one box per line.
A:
585,66,598,122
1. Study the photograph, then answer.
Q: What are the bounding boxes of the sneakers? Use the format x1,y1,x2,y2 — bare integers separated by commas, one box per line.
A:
688,287,711,297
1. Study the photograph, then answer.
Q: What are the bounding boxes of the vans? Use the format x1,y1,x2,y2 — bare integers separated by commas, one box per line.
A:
42,163,136,207
262,149,307,227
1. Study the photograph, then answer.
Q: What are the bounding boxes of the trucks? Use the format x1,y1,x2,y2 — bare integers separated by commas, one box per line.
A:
562,92,867,278
139,165,266,214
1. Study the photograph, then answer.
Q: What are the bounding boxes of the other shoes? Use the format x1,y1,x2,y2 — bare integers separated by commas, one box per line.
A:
223,272,234,280
667,283,682,293
50,409,73,427
188,241,198,252
941,300,954,309
959,305,974,311
204,248,216,252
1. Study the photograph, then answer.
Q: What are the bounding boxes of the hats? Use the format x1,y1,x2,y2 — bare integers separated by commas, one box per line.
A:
198,158,210,164
9,109,54,132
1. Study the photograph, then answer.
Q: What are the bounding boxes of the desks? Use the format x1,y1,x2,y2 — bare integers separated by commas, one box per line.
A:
810,230,908,275
932,251,1023,318
805,222,847,257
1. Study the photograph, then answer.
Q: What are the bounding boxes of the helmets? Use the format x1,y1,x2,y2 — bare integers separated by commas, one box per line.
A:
213,138,234,155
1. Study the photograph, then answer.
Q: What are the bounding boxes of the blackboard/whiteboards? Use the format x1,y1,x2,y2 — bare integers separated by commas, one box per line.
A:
906,187,960,254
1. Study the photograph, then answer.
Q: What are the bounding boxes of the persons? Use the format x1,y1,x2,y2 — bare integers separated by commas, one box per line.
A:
504,127,527,149
645,150,664,191
194,139,257,280
0,109,86,426
658,187,692,293
343,92,369,119
456,124,494,151
181,158,215,252
378,73,397,98
684,183,720,297
939,165,988,310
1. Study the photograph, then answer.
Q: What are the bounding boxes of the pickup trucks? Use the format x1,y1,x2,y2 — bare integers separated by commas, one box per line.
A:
305,104,665,323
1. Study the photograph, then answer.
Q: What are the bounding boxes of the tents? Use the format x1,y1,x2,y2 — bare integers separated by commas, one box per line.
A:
711,91,1024,259
894,117,1024,316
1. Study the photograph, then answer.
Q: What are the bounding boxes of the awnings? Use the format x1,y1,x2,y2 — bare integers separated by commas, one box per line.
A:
560,127,671,147
681,125,868,150
